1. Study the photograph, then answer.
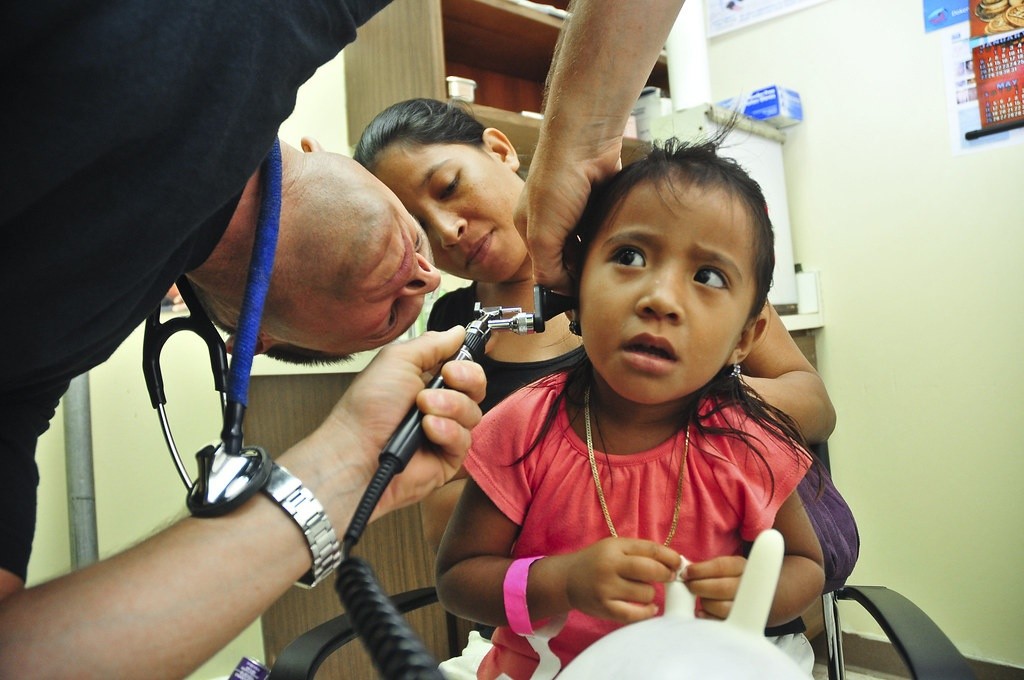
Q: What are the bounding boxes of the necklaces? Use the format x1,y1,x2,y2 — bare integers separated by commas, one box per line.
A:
578,378,692,551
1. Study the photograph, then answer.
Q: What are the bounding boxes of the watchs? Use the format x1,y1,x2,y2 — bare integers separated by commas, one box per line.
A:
254,460,343,588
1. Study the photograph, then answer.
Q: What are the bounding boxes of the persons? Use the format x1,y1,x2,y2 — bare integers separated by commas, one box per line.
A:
432,144,823,680
350,97,860,645
1,1,690,677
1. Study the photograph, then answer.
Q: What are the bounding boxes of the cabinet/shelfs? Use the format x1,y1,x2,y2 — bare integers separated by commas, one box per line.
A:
344,0,672,176
